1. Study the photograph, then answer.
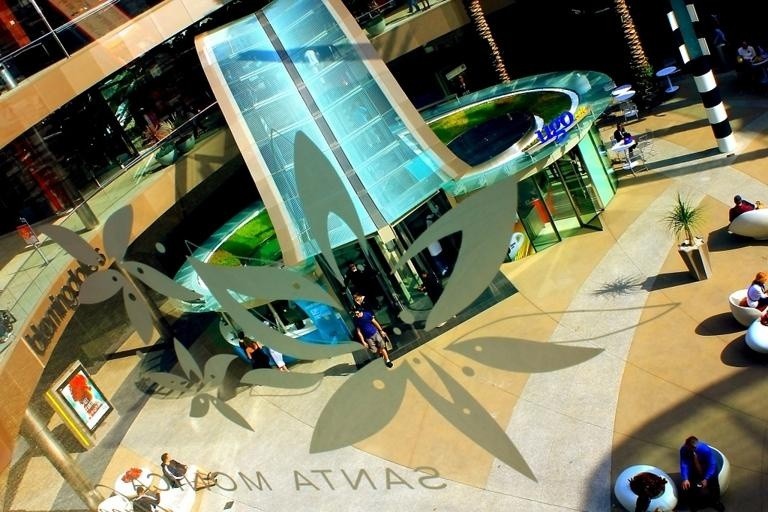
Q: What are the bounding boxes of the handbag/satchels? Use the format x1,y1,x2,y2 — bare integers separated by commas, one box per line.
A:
385,336,393,351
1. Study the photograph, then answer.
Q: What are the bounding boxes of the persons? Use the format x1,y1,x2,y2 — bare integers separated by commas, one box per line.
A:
709,29,727,58
304,50,323,73
407,0,420,12
421,213,449,302
350,101,383,150
342,262,393,368
421,0,430,10
729,195,755,223
184,101,209,139
614,124,638,153
736,41,756,95
680,436,725,512
133,487,160,512
239,331,290,373
161,453,188,488
747,272,768,308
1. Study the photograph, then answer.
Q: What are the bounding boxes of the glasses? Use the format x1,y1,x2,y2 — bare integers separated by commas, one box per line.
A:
354,313,359,315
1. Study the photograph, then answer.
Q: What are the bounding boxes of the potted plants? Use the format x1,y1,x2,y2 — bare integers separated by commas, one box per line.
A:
142,112,196,167
658,189,713,281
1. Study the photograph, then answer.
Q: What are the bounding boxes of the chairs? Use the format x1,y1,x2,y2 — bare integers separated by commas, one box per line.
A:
95,463,198,511
610,133,648,178
728,206,768,242
614,445,732,512
729,289,768,355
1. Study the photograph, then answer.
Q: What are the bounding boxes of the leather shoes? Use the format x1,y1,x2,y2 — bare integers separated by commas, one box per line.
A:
386,362,393,368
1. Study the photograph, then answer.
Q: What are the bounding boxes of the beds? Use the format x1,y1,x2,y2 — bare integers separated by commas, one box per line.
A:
656,66,679,94
